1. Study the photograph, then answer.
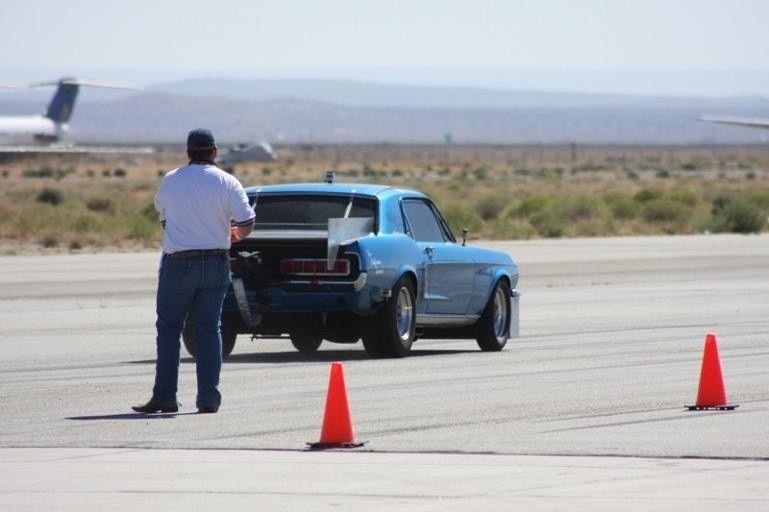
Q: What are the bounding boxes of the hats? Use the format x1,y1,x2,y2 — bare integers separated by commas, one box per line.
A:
187,129,215,150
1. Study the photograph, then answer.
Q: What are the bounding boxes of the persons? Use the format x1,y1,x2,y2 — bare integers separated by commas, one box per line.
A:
132,128,256,413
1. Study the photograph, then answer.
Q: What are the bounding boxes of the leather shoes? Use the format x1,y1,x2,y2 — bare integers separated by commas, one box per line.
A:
199,408,218,412
132,403,178,413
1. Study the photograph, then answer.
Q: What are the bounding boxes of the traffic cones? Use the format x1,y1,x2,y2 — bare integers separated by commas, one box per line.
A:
684,334,740,410
306,363,368,448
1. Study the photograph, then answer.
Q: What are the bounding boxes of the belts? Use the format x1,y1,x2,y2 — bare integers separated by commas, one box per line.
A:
172,250,230,256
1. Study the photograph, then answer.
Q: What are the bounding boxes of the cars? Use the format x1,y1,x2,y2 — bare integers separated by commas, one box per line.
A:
184,182,519,358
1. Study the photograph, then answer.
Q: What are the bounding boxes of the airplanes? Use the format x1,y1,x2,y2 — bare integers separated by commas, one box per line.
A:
0,77,144,141
